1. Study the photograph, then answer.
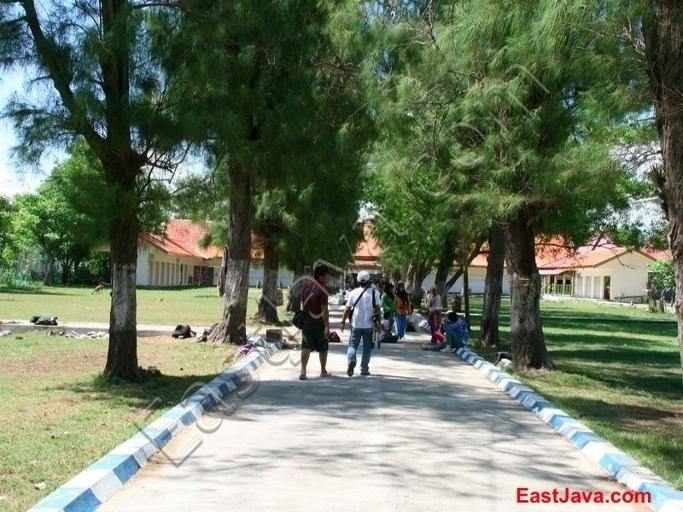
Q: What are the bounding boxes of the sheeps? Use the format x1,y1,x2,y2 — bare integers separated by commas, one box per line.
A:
91,285,104,296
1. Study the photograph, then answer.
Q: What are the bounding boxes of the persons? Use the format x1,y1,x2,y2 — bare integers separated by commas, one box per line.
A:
338,292,347,305
339,269,383,376
368,278,468,353
298,264,332,380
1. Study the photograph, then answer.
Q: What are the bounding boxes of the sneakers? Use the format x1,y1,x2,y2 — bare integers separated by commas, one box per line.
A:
451,347,457,353
421,344,430,350
347,360,356,377
361,371,370,375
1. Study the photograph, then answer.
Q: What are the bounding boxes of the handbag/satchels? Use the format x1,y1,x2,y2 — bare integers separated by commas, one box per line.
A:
348,310,354,322
293,312,310,328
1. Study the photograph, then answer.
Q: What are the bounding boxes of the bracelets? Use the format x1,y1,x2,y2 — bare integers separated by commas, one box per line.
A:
341,321,345,324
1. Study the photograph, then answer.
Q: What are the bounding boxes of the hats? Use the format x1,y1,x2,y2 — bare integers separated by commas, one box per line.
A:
357,270,370,282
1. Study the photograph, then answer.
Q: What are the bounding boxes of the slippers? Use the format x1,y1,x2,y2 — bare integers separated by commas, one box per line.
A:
320,371,331,376
298,374,306,379
